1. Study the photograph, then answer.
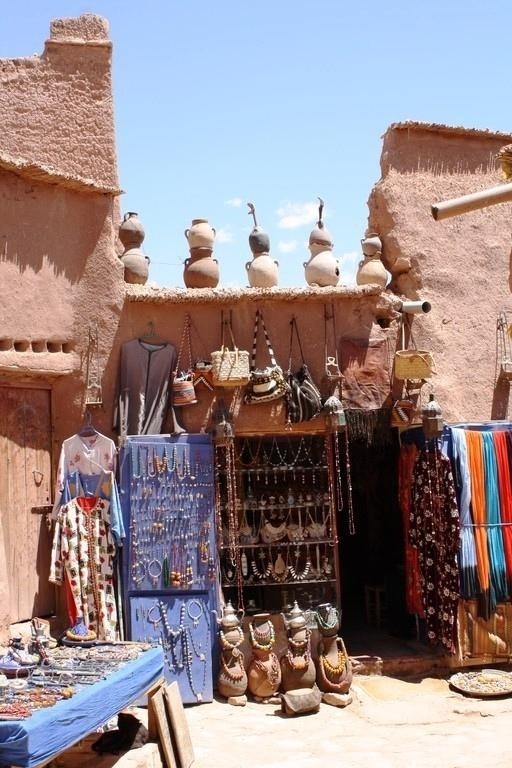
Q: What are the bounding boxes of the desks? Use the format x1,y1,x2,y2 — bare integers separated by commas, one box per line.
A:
0,640,165,768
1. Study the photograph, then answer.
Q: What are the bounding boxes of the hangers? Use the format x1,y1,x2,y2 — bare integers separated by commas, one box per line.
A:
74,479,98,510
78,409,97,435
66,451,105,475
139,321,168,344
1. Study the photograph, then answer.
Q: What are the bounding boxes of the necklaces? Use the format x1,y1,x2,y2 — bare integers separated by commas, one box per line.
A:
249,544,311,582
215,608,355,688
262,508,291,535
334,423,343,512
160,599,209,700
163,556,169,587
344,425,355,535
229,438,328,466
131,445,201,480
222,557,237,584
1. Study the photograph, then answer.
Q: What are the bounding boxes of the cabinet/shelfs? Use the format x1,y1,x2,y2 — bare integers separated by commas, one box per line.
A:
215,423,342,628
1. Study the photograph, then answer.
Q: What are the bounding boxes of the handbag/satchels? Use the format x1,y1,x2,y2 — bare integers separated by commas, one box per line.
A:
172,349,438,431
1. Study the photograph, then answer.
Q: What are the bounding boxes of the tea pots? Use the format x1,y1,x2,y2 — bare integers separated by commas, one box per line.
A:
211,602,245,626
280,601,310,629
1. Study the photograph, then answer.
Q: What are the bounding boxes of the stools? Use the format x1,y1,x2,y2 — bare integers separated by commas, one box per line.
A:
364,584,394,632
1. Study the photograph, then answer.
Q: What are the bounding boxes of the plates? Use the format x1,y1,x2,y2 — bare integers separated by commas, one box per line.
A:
61,636,97,647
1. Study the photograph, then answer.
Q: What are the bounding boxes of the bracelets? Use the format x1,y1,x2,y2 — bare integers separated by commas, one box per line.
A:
148,603,162,631
209,558,215,582
131,561,146,585
201,542,208,564
148,560,161,586
187,561,194,584
